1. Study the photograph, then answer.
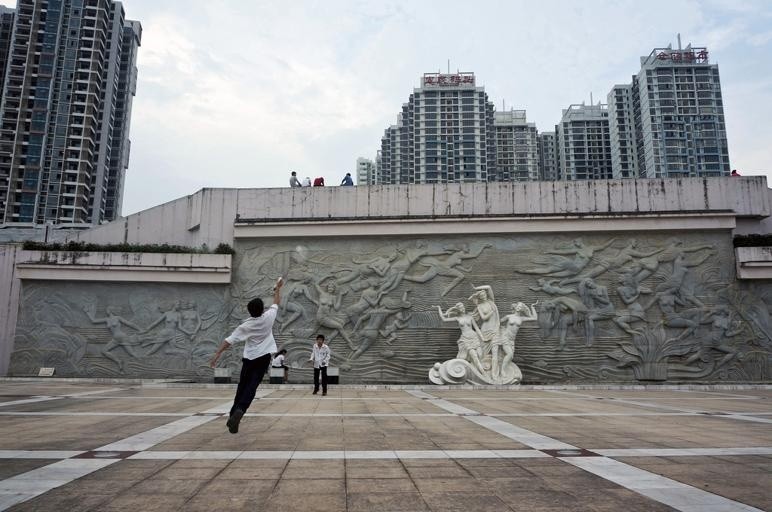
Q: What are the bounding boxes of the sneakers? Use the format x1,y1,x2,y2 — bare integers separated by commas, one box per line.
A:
313,387,328,395
226,408,244,434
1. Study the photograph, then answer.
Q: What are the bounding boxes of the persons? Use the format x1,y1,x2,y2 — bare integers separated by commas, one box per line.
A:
210,277,283,434
272,349,289,384
307,334,330,396
731,169,740,177
290,171,324,187
278,237,745,381
340,173,353,186
